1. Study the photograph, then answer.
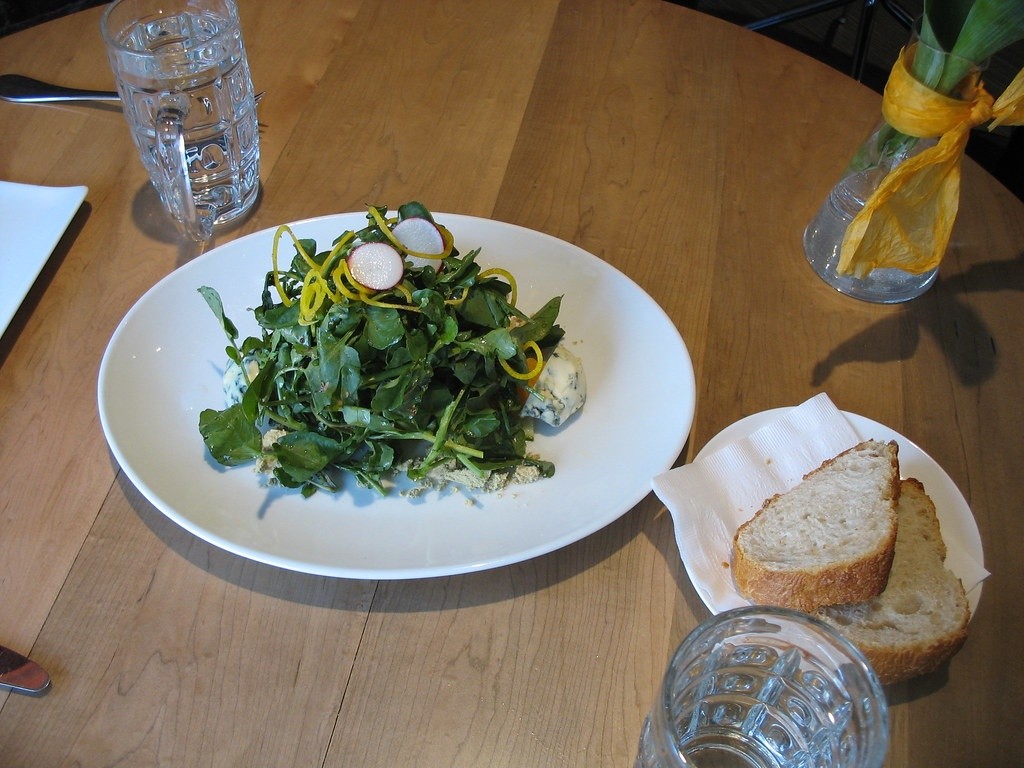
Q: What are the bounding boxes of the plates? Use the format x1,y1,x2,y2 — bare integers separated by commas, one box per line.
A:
679,405,985,628
98,208,697,582
0,179,89,341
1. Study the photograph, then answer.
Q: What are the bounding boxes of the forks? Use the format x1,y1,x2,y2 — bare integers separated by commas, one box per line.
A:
0,74,265,113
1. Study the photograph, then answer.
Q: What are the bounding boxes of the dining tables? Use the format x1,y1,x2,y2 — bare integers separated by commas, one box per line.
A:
0,0,1024,768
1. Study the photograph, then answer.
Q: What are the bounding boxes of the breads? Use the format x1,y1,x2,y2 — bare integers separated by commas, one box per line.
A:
800,477,973,683
732,438,900,611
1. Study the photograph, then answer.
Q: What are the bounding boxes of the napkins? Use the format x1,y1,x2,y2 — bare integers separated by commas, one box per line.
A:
649,391,991,667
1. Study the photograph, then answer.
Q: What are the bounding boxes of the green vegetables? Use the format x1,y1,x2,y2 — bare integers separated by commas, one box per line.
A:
196,198,567,500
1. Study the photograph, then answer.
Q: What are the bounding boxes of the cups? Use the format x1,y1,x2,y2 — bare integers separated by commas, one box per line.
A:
100,0,263,242
634,603,891,767
